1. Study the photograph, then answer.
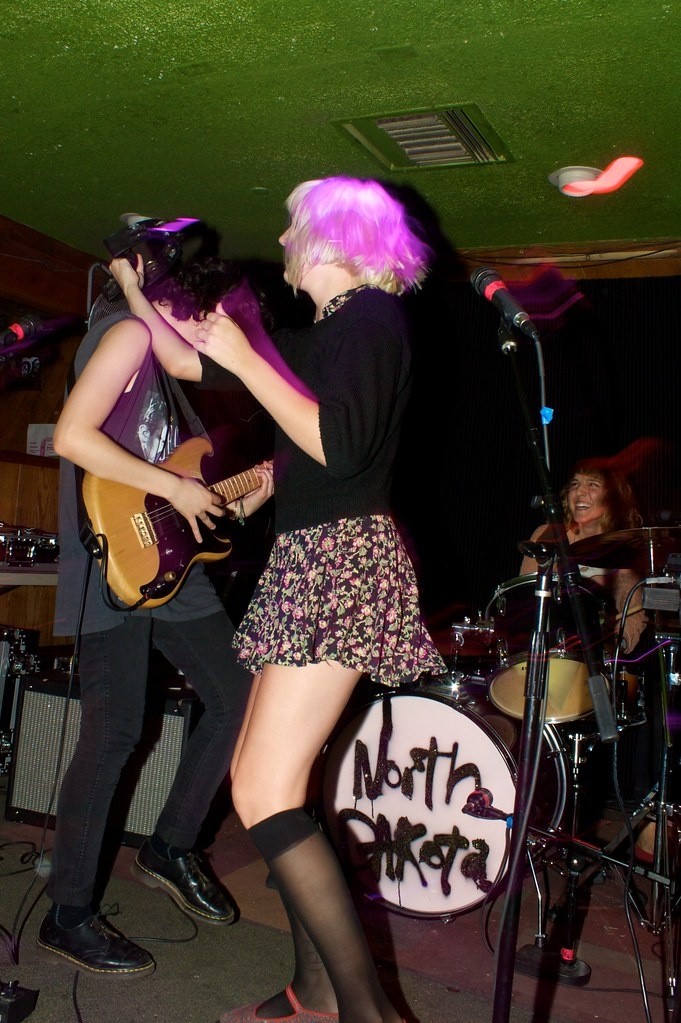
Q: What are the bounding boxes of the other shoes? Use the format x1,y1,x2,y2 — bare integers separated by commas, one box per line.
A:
220,981,339,1023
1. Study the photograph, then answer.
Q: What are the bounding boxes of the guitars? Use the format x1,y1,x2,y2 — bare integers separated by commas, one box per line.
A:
77,435,276,611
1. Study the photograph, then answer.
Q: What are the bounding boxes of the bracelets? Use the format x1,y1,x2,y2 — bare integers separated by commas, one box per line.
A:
229,497,247,526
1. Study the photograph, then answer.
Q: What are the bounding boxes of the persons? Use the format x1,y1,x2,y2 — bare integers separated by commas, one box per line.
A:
196,175,447,1022
520,455,650,654
34,216,276,980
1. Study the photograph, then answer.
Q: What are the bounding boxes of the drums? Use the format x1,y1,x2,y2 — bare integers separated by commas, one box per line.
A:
317,674,570,920
485,571,611,725
582,665,649,728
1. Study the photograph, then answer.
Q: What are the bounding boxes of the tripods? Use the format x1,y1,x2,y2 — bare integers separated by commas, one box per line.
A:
549,641,681,933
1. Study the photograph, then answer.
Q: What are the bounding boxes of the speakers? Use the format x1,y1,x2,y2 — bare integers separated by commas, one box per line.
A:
6,669,202,850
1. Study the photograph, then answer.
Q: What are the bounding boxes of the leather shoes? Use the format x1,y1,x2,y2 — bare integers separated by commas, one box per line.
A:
36,902,155,980
131,837,235,925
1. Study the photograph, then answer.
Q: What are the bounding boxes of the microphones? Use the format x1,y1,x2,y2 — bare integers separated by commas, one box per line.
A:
0,314,41,349
468,267,540,340
461,788,493,813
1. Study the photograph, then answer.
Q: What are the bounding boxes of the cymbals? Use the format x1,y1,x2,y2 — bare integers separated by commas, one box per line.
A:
565,525,681,571
